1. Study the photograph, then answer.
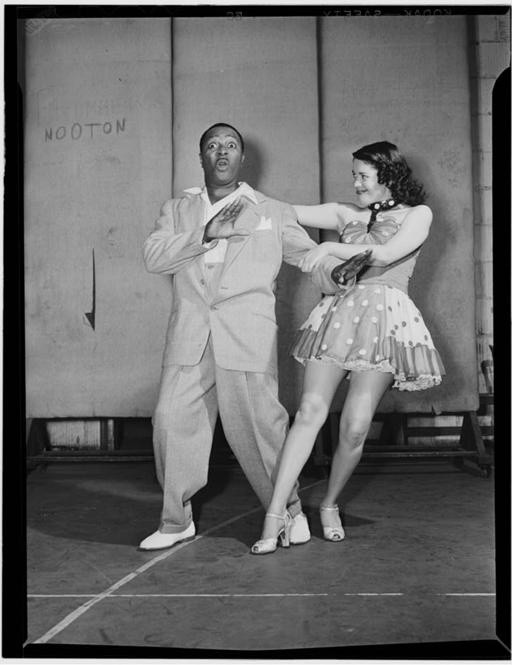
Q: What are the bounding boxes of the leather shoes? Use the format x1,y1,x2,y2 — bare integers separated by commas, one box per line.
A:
139,521,196,550
290,511,311,543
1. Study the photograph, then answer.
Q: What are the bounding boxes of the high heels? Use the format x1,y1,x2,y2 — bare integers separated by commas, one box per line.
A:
319,504,345,542
250,509,292,554
